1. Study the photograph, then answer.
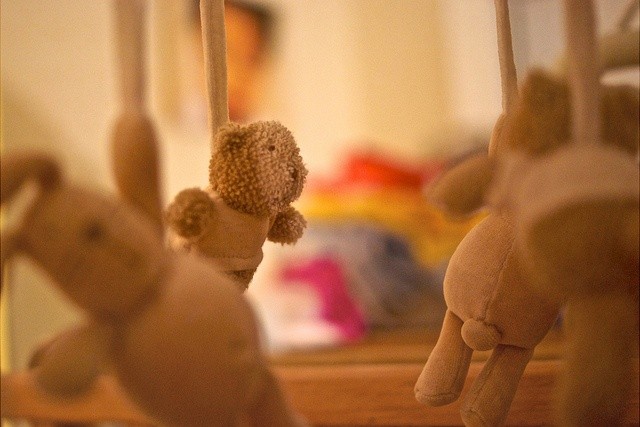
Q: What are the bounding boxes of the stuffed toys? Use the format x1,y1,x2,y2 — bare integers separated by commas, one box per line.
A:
0,116,300,427
414,70,640,427
167,121,309,294
493,143,639,427
